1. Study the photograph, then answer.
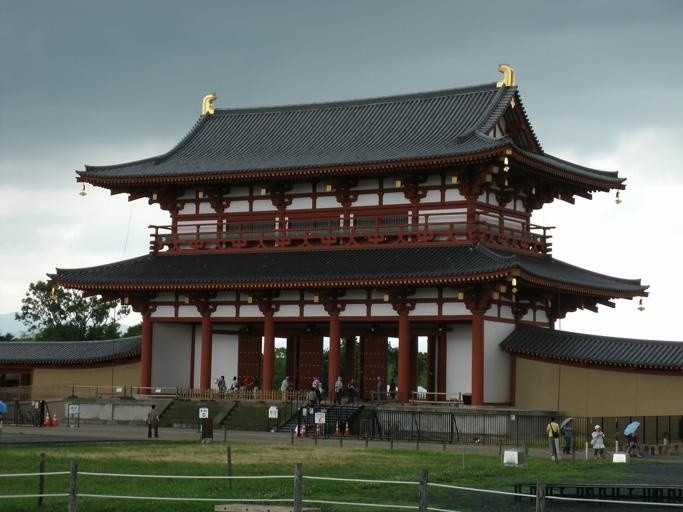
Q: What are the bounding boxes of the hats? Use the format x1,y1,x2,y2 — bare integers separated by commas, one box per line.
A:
595,425,601,429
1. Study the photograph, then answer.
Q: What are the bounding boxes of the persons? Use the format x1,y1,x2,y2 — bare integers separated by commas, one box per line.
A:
545,416,560,457
146,404,159,438
659,432,670,457
213,374,398,405
626,432,642,457
590,424,606,460
624,432,643,457
560,422,573,455
294,405,328,438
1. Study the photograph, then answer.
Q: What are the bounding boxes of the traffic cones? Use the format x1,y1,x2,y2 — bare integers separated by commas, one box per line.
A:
294,424,298,437
43,412,50,426
343,421,351,436
51,412,57,427
316,423,321,436
300,424,307,436
332,420,342,437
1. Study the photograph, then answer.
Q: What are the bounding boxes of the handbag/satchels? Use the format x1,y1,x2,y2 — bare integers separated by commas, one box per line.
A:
591,438,596,445
560,429,565,435
553,432,558,438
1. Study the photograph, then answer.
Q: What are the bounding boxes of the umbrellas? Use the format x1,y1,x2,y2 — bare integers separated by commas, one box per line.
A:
559,417,575,429
623,420,640,436
